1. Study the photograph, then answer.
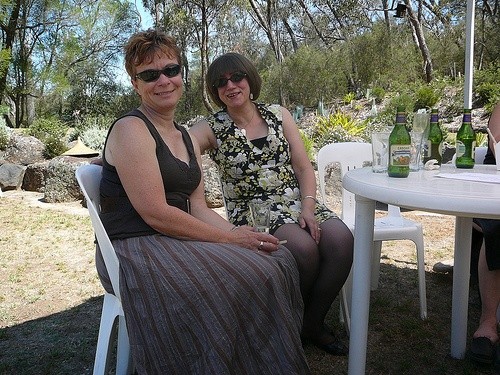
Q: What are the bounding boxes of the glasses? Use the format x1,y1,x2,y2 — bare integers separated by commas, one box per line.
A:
135,63,182,82
212,72,247,88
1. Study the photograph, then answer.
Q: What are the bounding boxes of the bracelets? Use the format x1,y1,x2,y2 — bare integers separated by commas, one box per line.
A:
231,226,239,232
301,195,317,205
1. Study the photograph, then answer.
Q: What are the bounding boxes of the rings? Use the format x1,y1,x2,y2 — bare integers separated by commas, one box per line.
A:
318,228,322,231
258,241,263,250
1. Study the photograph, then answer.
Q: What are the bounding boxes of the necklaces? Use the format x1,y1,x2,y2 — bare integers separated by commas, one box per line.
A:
229,104,254,137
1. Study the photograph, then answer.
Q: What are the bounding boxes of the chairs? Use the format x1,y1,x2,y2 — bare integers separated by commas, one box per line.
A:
319,142,428,328
70,161,130,375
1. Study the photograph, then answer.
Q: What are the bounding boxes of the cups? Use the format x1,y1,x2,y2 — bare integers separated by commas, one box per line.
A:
407,128,425,171
248,201,271,235
493,142,500,171
371,130,390,173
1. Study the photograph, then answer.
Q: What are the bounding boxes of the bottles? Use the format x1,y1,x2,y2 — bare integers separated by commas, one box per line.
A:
388,104,411,177
456,109,476,168
424,109,442,166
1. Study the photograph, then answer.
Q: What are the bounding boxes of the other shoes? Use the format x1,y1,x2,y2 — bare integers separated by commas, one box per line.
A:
300,322,349,356
471,336,498,365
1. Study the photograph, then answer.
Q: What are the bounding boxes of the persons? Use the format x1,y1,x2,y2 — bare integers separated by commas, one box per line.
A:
467,101,500,356
185,51,354,358
94,32,312,375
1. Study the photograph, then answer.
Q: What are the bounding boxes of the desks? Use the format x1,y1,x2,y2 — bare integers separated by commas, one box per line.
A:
343,163,500,375
